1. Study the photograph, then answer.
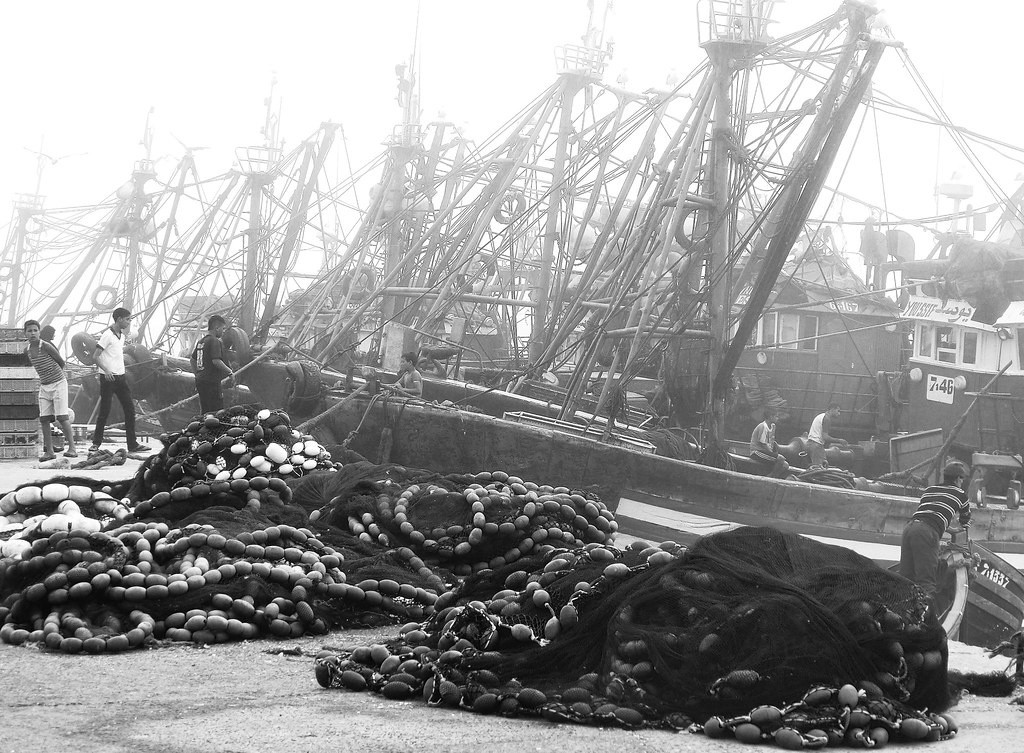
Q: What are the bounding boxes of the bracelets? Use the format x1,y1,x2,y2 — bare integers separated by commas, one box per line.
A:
227,371,233,376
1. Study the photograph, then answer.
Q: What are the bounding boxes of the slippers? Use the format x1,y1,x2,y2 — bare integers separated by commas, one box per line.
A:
39,453,56,462
64,449,78,457
128,445,151,452
89,444,100,451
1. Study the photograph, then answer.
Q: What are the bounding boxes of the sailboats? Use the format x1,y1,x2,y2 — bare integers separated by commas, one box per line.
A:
0,0,1023,557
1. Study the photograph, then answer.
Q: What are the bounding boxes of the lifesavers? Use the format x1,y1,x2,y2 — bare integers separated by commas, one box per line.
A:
674,203,708,252
457,254,497,294
676,248,709,297
283,358,323,417
493,192,529,224
90,285,118,310
122,343,155,401
222,326,251,366
71,332,98,366
342,265,376,300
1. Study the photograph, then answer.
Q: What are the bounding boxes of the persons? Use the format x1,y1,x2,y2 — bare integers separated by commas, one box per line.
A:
38,325,60,354
23,319,79,462
189,315,235,415
805,404,848,469
749,408,789,480
89,307,152,452
899,461,972,597
393,352,423,399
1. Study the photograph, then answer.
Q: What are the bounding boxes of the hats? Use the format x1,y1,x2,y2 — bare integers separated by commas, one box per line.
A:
944,461,966,478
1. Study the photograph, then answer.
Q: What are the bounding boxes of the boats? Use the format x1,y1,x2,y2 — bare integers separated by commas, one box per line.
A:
945,513,1024,658
881,541,970,640
